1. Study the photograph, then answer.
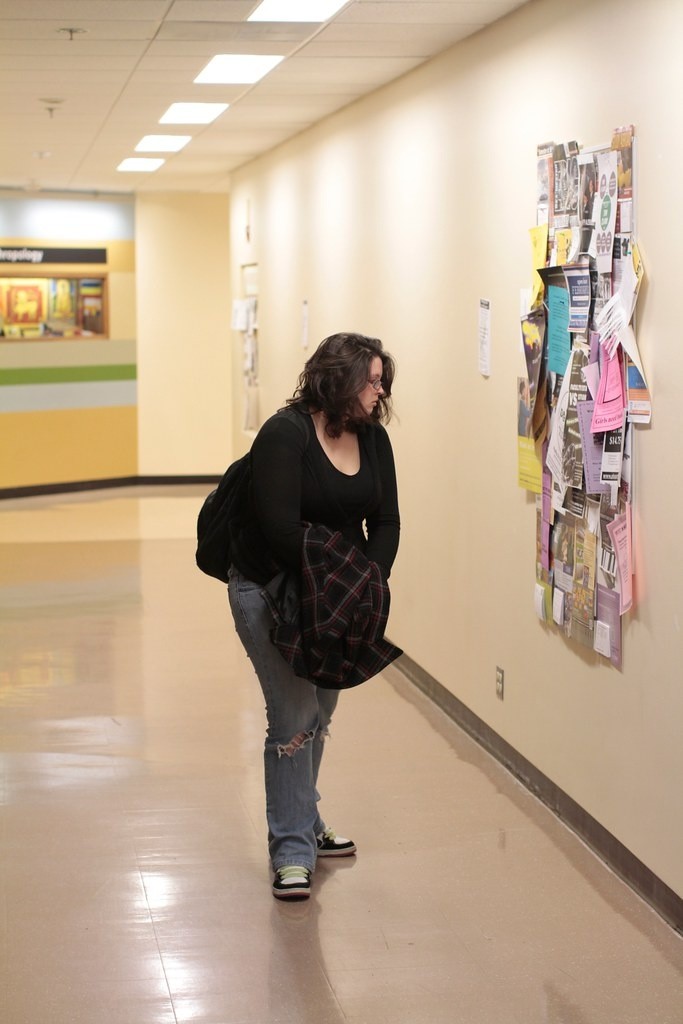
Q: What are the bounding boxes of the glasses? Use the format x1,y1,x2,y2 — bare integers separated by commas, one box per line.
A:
368,379,382,390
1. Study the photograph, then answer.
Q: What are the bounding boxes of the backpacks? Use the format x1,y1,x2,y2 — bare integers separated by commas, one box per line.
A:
196,406,313,584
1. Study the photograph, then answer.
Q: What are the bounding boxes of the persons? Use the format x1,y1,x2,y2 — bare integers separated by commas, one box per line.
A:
228,332,403,900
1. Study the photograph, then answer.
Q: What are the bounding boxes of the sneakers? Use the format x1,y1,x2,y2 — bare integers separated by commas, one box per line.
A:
316,828,357,856
272,865,311,899
